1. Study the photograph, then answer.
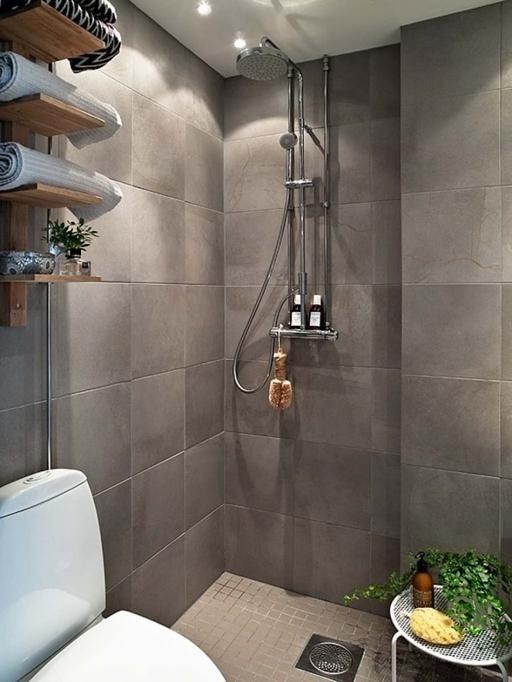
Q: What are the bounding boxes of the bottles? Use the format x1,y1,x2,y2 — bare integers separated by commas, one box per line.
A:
412,559,434,608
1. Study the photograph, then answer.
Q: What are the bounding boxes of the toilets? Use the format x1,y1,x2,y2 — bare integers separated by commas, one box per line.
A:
0,466,227,682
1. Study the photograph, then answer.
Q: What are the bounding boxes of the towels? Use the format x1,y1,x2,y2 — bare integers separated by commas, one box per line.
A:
0,50,122,151
0,141,123,227
0,0,123,75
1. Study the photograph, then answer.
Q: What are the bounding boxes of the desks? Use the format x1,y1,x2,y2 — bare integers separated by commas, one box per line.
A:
387,583,512,682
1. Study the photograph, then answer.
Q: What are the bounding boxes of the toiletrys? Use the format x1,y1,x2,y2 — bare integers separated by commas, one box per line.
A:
306,293,326,330
289,293,303,329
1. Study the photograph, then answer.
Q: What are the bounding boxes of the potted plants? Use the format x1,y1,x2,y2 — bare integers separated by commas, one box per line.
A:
343,543,512,652
42,212,100,275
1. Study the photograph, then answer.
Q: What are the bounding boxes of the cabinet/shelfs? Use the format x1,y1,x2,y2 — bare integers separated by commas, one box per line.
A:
0,1,106,331
